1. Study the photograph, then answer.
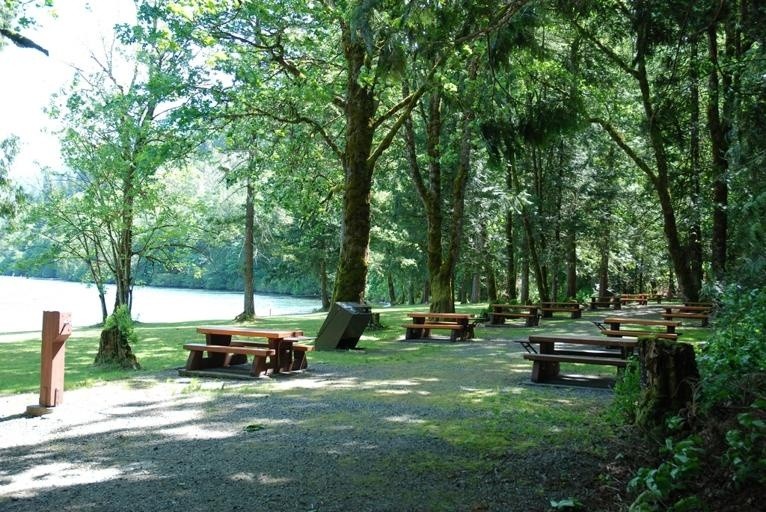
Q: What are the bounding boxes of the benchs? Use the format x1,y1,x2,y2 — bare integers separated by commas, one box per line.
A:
228,340,316,370
487,313,543,326
402,321,475,342
540,307,584,319
183,343,276,377
660,302,712,328
602,330,682,351
587,295,665,310
512,339,636,383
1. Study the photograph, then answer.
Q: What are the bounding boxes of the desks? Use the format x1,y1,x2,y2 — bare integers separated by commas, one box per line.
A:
406,312,474,342
540,302,579,319
491,304,541,326
603,315,681,338
528,335,638,383
195,325,304,378
